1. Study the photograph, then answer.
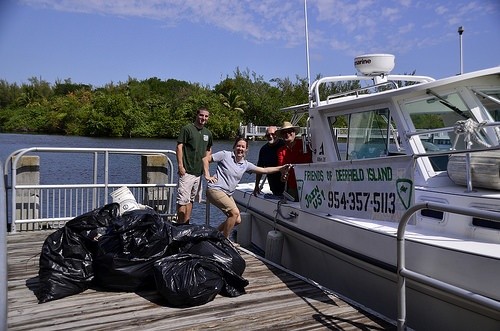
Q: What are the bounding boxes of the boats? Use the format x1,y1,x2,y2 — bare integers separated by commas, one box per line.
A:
233,54,499,331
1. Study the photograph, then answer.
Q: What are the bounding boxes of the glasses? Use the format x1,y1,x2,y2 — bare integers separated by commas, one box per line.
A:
282,129,294,134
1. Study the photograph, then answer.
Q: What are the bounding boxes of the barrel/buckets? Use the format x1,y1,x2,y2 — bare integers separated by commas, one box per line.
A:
109,186,154,216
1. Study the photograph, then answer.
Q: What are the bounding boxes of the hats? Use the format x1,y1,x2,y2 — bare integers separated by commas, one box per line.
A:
275,121,300,137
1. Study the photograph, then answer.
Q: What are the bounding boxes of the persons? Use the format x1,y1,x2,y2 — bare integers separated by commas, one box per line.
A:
253,126,286,198
202,137,292,247
176,107,213,223
275,120,312,203
